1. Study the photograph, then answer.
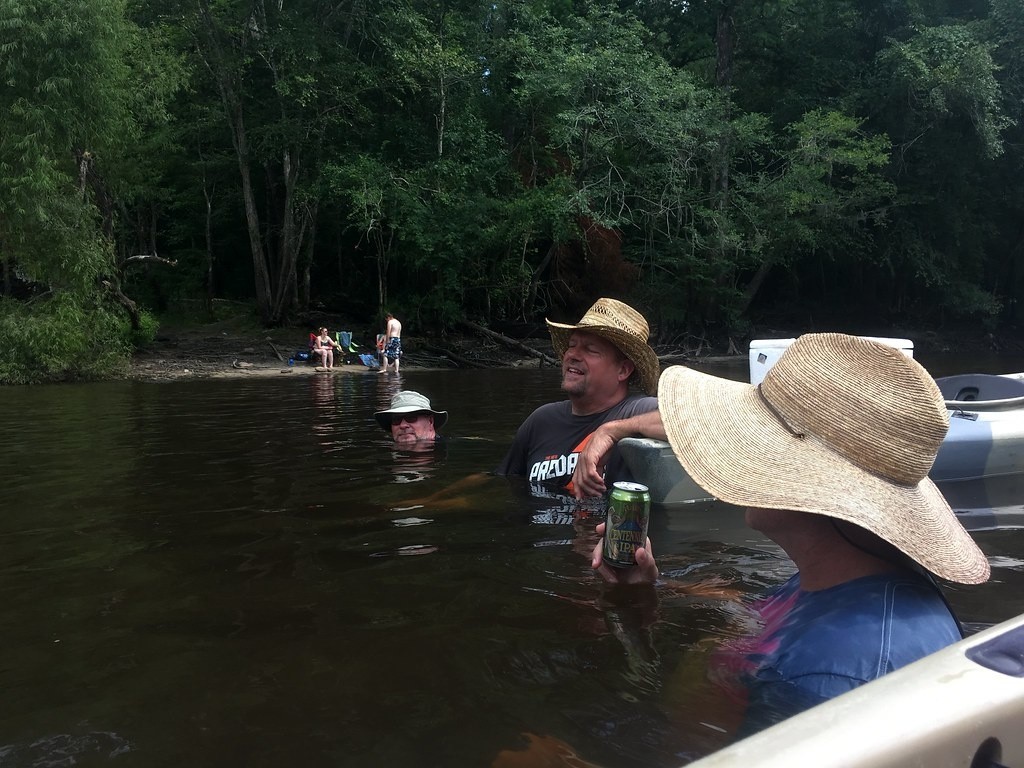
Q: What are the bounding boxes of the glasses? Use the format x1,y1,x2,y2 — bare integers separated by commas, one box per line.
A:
391,414,418,424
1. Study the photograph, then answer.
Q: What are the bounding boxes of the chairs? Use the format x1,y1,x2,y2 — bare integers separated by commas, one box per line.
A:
377,334,383,361
309,332,359,365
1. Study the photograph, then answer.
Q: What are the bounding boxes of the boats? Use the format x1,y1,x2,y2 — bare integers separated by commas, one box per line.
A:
680,613,1024,768
617,337,1024,508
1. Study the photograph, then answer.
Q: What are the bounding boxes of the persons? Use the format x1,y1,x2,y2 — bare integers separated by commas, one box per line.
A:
488,332,992,768
376,313,402,378
375,390,449,440
498,298,668,501
312,326,342,369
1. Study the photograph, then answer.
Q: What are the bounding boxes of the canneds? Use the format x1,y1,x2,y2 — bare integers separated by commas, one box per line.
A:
602,481,650,569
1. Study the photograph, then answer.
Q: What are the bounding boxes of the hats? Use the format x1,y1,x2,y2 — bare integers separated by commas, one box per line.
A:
657,332,991,586
545,298,659,397
374,391,448,431
